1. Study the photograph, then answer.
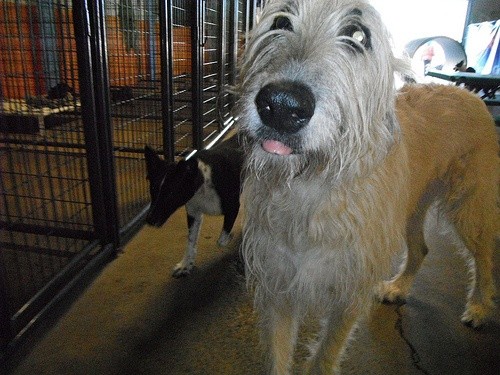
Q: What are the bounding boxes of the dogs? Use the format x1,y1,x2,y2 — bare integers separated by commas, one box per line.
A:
144,129,253,279
226,0,500,375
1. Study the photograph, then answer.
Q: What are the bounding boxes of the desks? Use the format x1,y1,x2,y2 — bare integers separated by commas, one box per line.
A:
0,95,81,133
